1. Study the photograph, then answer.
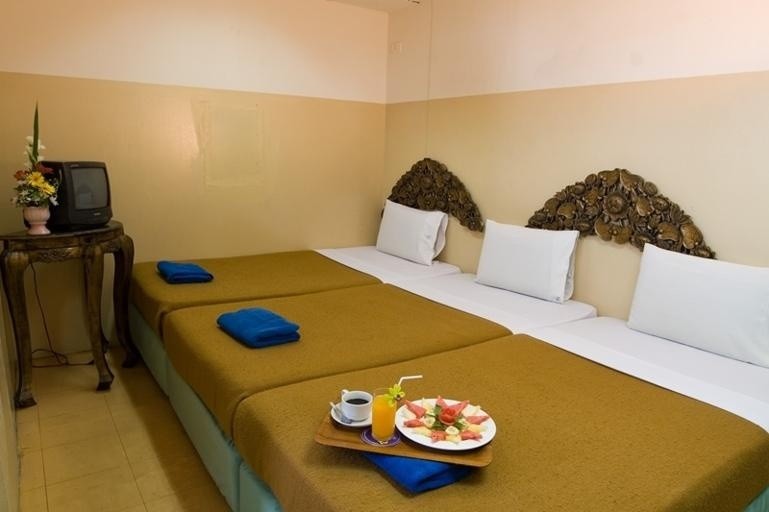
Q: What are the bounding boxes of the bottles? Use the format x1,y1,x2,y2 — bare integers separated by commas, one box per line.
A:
372,388,398,442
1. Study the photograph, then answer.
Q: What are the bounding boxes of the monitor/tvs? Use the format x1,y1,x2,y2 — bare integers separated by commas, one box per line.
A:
22,161,114,229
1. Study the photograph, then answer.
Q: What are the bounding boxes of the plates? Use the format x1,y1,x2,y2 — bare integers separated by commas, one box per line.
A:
396,397,499,453
331,400,373,427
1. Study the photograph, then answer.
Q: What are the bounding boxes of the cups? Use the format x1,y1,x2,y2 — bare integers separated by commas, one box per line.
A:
339,388,374,420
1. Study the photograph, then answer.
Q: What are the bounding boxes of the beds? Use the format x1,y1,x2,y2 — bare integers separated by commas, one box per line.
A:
126,157,486,399
232,242,769,511
161,169,715,511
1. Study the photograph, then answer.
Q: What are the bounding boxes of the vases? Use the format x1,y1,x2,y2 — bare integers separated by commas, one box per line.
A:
23,205,52,236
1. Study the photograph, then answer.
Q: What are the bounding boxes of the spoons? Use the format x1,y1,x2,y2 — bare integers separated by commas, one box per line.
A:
327,401,351,423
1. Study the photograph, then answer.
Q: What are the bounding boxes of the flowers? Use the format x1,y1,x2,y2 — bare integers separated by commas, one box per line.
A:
7,100,60,209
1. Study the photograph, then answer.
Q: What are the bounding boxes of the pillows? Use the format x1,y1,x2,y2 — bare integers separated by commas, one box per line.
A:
373,197,448,266
626,241,769,370
474,218,580,305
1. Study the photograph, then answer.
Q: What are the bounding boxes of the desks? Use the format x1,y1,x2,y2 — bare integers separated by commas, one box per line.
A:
1,219,138,409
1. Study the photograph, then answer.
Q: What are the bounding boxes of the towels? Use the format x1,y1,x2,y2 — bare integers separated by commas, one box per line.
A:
216,308,301,348
157,260,214,284
363,449,477,495
